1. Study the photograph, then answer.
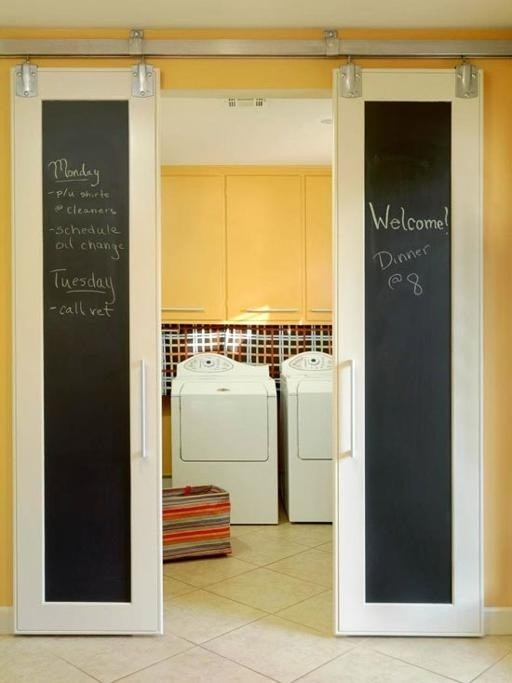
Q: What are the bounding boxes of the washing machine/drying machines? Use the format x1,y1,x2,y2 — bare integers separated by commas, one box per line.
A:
280,350,338,524
168,353,281,525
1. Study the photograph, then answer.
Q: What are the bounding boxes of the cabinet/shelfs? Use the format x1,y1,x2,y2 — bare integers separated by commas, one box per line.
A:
159,163,333,326
